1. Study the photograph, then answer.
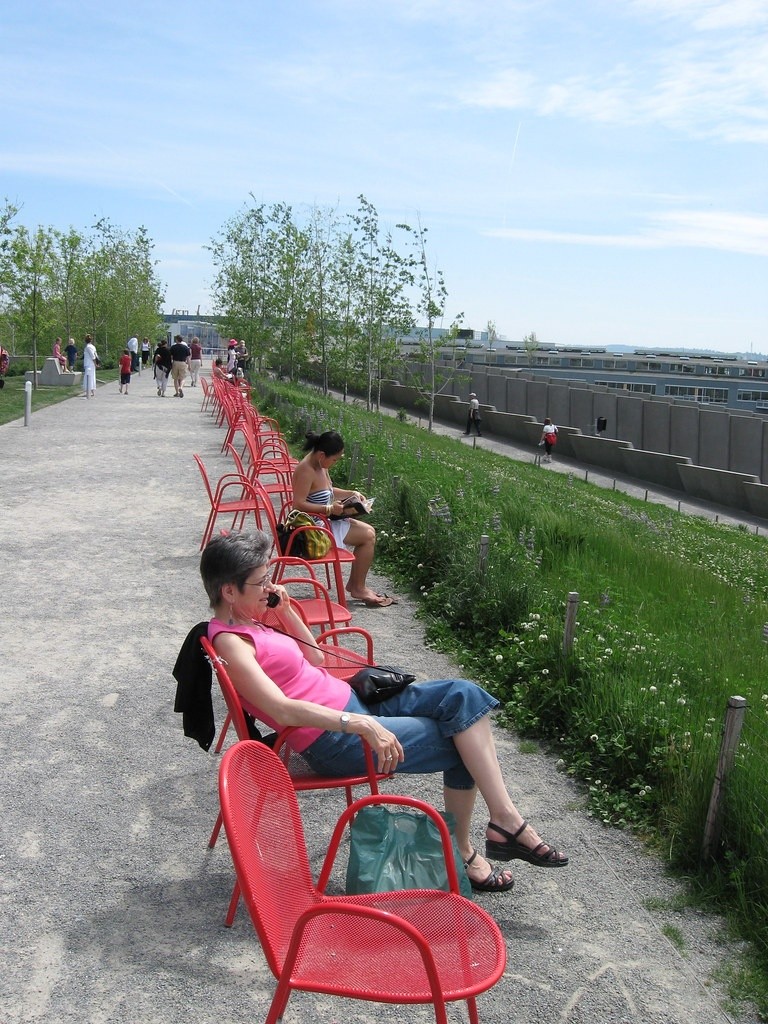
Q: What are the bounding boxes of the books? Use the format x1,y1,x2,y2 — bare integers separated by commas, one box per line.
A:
329,496,375,521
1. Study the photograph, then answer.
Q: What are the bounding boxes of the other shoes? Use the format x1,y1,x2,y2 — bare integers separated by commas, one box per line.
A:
119,388,122,393
462,432,470,435
157,388,161,396
160,392,165,397
191,381,195,386
193,384,198,387
63,368,69,373
124,391,128,395
543,456,552,463
173,392,179,397
477,434,482,436
178,388,184,398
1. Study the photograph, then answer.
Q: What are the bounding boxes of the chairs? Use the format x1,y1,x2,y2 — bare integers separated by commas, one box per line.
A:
219,739,506,1024
200,636,395,928
195,359,376,682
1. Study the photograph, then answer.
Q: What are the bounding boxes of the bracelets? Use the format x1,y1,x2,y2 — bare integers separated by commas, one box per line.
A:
325,505,331,516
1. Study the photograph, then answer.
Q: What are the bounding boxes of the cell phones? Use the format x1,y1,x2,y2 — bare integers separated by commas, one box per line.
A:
267,592,281,608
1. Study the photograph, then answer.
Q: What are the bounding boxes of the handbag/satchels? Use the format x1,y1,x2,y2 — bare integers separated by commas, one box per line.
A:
95,354,102,365
348,664,417,705
545,432,556,445
343,805,474,906
276,510,332,561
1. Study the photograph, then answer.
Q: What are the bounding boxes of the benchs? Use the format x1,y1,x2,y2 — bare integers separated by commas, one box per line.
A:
25,357,82,386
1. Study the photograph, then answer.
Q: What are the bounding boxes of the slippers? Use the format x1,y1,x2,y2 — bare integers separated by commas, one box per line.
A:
352,592,399,608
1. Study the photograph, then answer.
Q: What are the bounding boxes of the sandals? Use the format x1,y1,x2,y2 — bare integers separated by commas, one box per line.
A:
464,848,515,892
485,819,569,868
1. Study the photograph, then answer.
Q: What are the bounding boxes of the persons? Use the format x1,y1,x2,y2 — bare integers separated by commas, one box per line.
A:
540,418,558,463
0,345,10,390
53,337,78,373
462,393,482,436
199,532,568,893
81,334,98,397
215,339,251,385
119,334,204,399
291,431,387,603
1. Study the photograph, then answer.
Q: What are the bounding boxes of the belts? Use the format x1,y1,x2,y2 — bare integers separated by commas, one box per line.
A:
174,360,186,362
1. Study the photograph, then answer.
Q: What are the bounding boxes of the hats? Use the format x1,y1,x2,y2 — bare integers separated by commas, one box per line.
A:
215,358,223,365
227,339,238,346
469,393,476,397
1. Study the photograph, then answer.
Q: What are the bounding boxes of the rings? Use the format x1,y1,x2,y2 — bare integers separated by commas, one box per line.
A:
386,754,393,762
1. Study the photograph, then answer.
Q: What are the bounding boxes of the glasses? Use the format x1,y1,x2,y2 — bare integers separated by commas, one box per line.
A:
243,566,274,590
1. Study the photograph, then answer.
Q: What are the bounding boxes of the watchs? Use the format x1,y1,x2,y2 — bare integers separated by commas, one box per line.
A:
340,711,351,734
